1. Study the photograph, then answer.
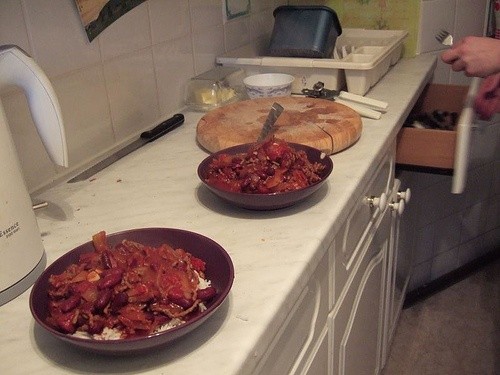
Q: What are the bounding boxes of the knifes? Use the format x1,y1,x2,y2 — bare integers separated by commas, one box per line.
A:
66,113,185,183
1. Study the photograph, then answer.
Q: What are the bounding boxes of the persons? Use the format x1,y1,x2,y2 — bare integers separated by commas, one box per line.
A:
442,36,500,121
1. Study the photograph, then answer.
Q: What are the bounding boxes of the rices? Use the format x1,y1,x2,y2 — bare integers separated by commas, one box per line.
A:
65,270,213,341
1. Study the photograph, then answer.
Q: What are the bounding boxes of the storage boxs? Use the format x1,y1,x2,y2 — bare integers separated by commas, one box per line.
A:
268,5,342,58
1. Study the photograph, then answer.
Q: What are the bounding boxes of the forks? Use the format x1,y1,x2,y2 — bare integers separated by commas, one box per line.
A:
434,30,455,49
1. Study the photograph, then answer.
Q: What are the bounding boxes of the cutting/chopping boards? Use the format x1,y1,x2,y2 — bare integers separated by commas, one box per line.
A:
196,96,363,156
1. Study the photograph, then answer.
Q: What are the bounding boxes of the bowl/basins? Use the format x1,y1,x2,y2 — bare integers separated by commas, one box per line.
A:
29,227,234,357
243,73,295,99
197,140,334,210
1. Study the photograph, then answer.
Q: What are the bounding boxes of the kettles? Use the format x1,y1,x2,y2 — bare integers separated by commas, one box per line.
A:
0,44,69,307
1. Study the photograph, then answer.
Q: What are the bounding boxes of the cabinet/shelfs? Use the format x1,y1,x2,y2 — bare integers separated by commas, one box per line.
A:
237,55,479,375
